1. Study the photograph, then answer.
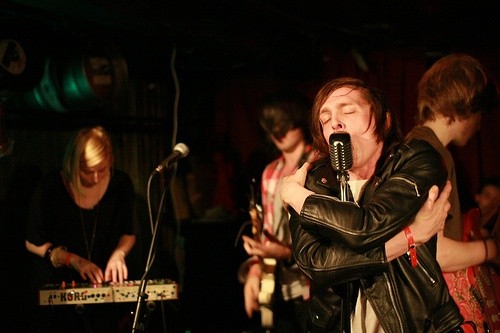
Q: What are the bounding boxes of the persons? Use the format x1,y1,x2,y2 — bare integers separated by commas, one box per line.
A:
241,95,327,333
280,76,465,333
23,125,139,286
401,54,498,333
465,181,500,242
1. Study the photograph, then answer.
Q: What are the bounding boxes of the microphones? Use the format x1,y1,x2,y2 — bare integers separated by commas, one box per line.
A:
151,143,189,177
329,132,354,202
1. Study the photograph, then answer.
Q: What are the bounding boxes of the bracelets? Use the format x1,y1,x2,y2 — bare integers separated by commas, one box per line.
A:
50,246,67,269
480,239,489,263
403,226,418,269
44,245,57,260
65,253,74,267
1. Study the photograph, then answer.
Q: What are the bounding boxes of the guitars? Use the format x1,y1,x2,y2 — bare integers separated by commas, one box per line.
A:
440,205,499,333
247,176,278,330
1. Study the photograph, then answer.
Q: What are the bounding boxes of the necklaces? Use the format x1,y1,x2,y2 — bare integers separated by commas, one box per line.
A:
77,178,101,261
